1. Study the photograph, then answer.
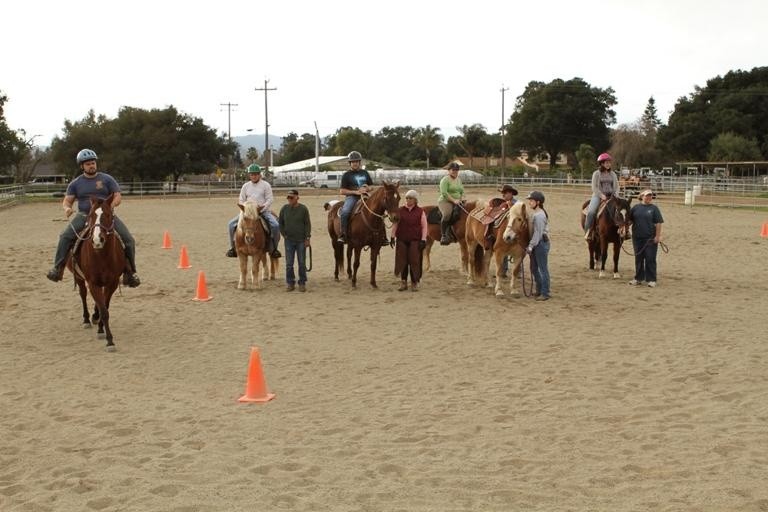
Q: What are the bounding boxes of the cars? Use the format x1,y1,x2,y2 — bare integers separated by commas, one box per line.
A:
299,177,315,187
672,166,730,188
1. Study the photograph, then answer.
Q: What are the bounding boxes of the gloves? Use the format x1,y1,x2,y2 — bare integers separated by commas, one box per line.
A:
525,246,532,253
389,238,394,248
418,240,425,251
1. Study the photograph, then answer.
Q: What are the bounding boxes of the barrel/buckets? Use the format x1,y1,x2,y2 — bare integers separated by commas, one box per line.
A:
685,191,694,205
694,186,702,197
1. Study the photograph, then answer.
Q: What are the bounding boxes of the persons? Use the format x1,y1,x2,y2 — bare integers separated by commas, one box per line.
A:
46,148,140,289
337,151,373,244
525,191,550,302
278,190,312,292
224,164,280,258
324,200,340,210
437,162,464,245
492,184,524,280
584,152,621,241
628,188,664,288
389,188,428,291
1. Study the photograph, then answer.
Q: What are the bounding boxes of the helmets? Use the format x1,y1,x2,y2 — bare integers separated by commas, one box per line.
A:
76,148,97,164
247,164,260,173
525,190,544,203
347,150,362,161
596,153,611,161
447,162,459,170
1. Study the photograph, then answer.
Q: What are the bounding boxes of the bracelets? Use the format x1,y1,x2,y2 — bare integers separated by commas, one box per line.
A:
304,237,310,240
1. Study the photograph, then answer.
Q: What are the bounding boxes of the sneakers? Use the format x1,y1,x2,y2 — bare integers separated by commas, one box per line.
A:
628,279,641,285
122,274,140,286
647,280,657,288
298,284,305,292
271,248,281,258
535,295,546,301
337,236,344,243
286,286,294,292
46,269,63,282
225,249,237,257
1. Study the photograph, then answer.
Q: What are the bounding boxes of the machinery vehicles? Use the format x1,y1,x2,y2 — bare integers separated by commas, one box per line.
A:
616,170,656,203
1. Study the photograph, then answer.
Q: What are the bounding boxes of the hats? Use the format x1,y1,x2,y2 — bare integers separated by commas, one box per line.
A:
497,184,518,195
639,189,652,196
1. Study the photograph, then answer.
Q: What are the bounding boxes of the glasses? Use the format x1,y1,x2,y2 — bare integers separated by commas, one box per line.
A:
501,191,506,194
286,195,294,199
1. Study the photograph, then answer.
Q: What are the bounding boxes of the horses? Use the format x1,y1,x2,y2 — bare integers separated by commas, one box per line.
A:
65,191,127,354
323,179,402,290
233,202,281,291
421,199,480,275
580,190,634,279
464,200,532,297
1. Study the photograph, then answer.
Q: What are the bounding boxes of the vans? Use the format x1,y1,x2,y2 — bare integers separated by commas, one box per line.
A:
315,174,342,188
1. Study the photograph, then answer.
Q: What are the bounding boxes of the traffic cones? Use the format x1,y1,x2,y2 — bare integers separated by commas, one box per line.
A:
178,246,193,270
191,269,215,304
760,222,768,237
160,231,174,249
239,345,277,406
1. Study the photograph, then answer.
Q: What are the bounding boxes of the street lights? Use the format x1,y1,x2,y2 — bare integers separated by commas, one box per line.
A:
497,86,510,182
245,125,271,132
219,101,239,142
254,79,278,185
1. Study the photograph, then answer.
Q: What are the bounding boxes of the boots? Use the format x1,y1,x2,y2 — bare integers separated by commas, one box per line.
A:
396,281,408,291
411,282,419,291
440,219,449,244
